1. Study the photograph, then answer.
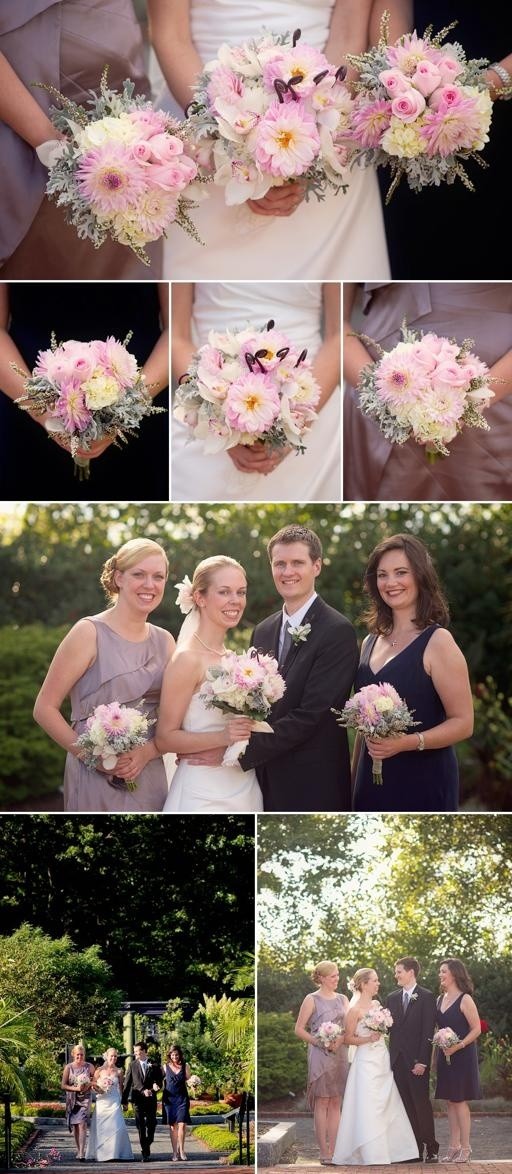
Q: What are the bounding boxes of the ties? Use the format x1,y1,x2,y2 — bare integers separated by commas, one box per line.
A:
274,619,299,674
142,1062,148,1078
402,991,412,1017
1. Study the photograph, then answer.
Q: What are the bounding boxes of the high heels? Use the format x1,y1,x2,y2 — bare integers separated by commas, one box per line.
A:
452,1145,473,1164
441,1142,463,1163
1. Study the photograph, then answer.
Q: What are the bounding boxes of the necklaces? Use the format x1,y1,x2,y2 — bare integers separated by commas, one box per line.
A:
443,992,459,1004
387,630,413,649
193,632,228,657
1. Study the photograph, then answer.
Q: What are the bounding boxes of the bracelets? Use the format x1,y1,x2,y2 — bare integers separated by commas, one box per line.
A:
460,1041,465,1049
414,730,424,752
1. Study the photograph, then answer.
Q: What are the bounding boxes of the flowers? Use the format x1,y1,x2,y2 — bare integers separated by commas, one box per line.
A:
343,8,512,205
328,681,423,785
409,992,418,1002
174,574,195,615
75,1074,90,1095
146,1062,152,1068
29,64,213,267
287,622,311,646
198,644,287,759
365,1007,394,1047
313,1021,343,1054
182,28,355,203
174,321,324,458
70,695,158,792
348,316,508,467
186,1074,202,1093
97,1077,114,1098
430,1026,463,1066
10,327,167,482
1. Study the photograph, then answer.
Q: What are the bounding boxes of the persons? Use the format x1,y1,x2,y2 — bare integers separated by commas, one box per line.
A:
32,536,176,812
331,967,420,1166
153,1045,198,1161
153,554,264,812
173,523,360,812
378,956,440,1164
85,1048,135,1162
60,1045,95,1161
122,1042,163,1163
433,958,482,1165
350,533,476,811
294,960,351,1166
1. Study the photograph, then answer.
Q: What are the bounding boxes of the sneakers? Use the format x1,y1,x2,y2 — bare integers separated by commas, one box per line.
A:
178,1146,188,1161
75,1150,80,1159
319,1149,341,1165
171,1151,179,1162
423,1143,440,1164
79,1151,86,1161
141,1148,150,1163
